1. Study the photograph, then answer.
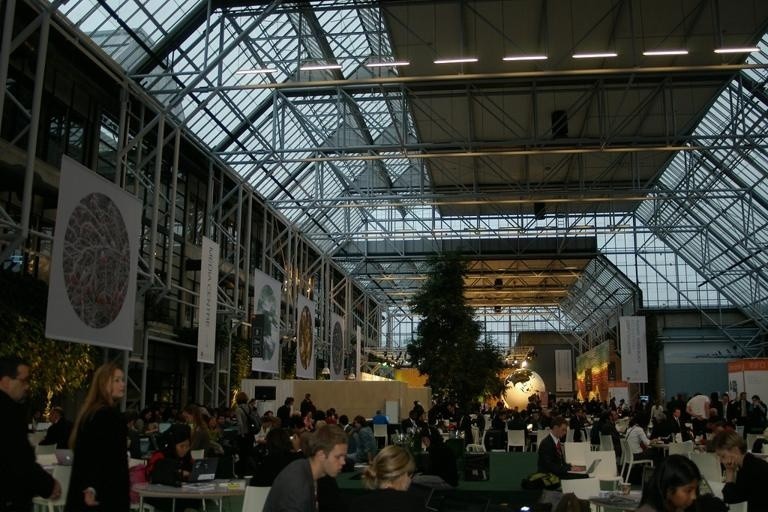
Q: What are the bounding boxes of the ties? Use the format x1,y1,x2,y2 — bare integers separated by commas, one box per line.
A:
557,441,562,456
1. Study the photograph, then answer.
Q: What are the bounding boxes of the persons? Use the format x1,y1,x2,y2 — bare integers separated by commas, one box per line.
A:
122,390,768,512
38,407,75,450
0,355,62,512
62,363,131,512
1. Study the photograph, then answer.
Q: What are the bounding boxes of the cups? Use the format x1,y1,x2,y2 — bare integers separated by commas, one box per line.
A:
599,491,610,499
664,438,669,444
622,483,631,496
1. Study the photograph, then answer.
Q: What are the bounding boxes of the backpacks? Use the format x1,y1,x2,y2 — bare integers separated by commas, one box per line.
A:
240,405,260,434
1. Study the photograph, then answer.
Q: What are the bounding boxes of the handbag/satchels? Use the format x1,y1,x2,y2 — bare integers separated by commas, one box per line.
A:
463,454,489,480
156,460,184,487
520,473,559,489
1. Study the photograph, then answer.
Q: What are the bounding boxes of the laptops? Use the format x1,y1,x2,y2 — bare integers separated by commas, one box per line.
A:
566,459,601,475
182,458,218,482
55,450,73,465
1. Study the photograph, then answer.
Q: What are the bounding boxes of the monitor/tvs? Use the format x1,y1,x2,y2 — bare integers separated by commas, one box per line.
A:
640,395,649,403
